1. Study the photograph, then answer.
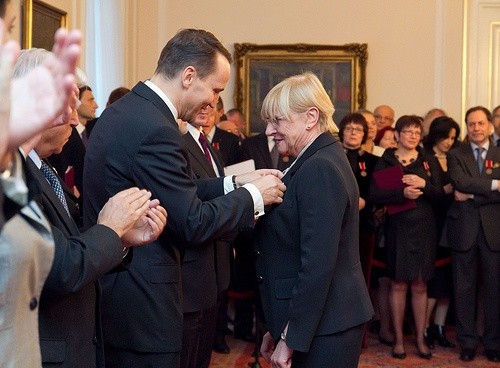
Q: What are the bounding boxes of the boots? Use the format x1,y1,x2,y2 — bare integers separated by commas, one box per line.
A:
432,321,456,348
424,327,436,349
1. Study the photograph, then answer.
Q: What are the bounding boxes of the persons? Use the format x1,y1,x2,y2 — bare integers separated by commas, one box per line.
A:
12,47,167,368
242,122,296,172
339,105,500,362
85,28,286,368
1,0,55,367
48,85,131,216
0,26,82,234
258,72,375,368
203,96,250,165
180,105,231,368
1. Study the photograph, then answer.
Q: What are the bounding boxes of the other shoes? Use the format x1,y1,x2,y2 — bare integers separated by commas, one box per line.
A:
212,336,230,353
391,341,406,359
417,345,433,359
377,331,396,346
483,349,500,362
234,332,255,343
458,348,476,362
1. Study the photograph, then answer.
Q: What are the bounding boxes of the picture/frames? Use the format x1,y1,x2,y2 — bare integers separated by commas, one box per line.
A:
21,0,69,52
232,42,370,136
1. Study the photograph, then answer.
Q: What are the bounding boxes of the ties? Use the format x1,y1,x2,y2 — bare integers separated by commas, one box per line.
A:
199,133,216,176
81,130,89,148
39,159,71,219
476,148,485,176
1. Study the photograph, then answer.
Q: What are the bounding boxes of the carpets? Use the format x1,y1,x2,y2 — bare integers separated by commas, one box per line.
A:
236,299,500,368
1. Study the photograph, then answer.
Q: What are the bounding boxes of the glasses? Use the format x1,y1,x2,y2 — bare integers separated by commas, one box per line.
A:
265,111,296,125
400,129,422,137
343,125,364,134
